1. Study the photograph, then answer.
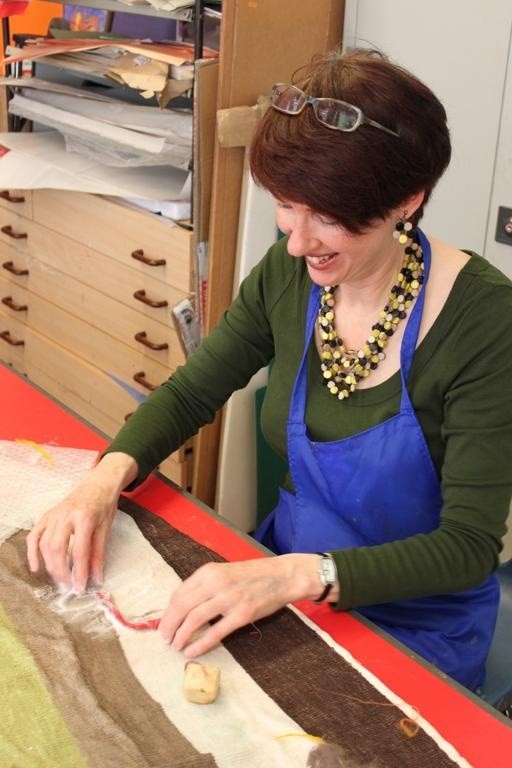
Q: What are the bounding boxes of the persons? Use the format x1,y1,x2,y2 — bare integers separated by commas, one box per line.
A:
24,50,509,695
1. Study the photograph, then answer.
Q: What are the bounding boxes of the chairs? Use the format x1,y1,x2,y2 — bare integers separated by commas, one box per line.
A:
214,364,269,538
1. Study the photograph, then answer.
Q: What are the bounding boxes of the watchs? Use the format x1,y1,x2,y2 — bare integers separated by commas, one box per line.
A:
314,550,338,603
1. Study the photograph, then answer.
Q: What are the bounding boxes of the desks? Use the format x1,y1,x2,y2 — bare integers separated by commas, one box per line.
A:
0,360,512,768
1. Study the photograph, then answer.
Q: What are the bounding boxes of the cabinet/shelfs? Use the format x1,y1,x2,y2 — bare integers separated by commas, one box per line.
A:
1,1,344,512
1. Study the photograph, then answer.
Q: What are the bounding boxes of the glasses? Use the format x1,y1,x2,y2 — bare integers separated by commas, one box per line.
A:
270,82,400,139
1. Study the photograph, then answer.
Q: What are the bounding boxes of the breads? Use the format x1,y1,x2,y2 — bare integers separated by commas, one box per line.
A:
183,662,221,705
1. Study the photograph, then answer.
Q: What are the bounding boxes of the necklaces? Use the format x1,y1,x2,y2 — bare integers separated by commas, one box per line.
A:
314,236,428,401
